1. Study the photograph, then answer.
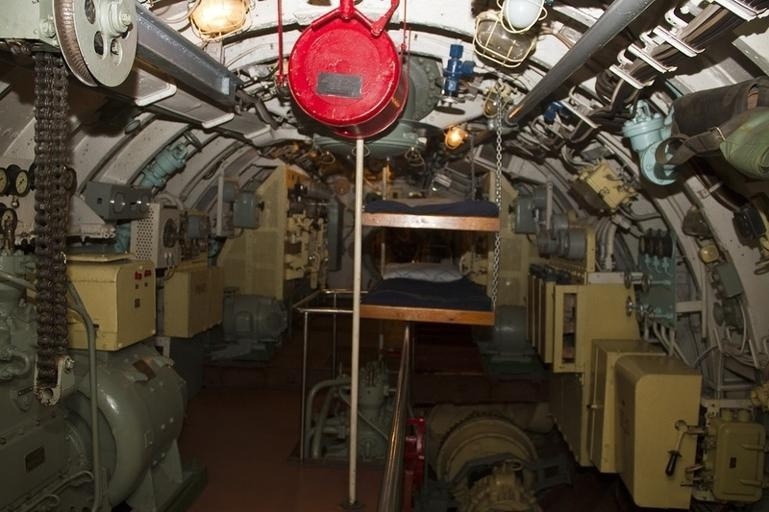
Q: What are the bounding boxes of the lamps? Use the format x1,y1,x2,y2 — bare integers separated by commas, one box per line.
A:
444,127,466,151
495,0,550,37
471,14,538,71
188,0,259,42
481,91,512,118
